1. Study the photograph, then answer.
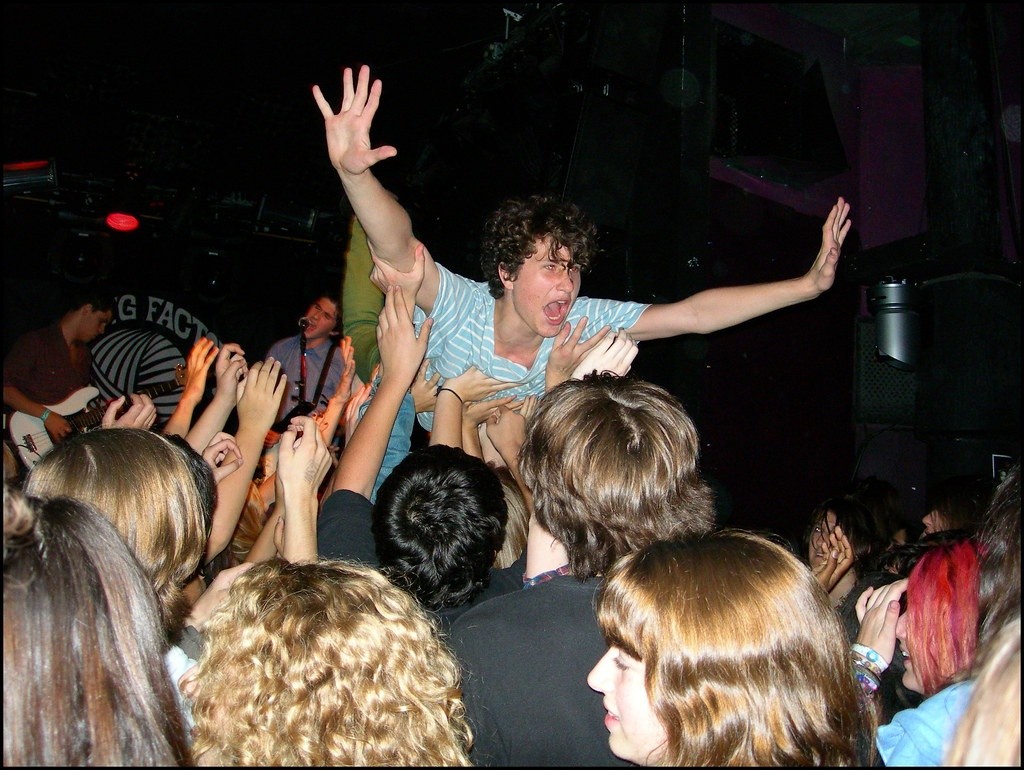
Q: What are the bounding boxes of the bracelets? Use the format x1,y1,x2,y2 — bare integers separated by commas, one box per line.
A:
433,386,463,405
850,642,890,697
41,409,51,422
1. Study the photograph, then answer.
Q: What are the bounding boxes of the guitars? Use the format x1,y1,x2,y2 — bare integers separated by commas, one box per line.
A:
5,365,209,472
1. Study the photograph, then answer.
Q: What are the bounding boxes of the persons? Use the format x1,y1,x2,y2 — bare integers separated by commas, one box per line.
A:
2,65,854,768
805,457,1024,768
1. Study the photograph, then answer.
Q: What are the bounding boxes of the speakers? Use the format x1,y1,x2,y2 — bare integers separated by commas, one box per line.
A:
855,271,1024,542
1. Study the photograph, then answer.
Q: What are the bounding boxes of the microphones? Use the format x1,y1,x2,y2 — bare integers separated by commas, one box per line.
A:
300,317,311,329
